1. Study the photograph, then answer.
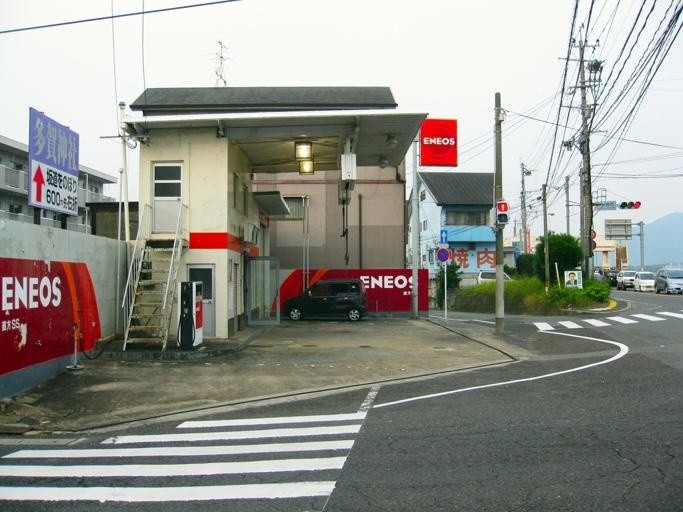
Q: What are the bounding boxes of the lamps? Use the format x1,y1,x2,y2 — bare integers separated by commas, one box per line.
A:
294,141,315,175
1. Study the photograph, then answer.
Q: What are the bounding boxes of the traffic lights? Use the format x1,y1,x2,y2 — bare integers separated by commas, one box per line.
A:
497,202,508,225
619,201,641,209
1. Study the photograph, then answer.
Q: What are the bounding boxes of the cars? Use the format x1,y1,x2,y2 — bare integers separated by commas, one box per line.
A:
594,267,683,295
284,279,367,321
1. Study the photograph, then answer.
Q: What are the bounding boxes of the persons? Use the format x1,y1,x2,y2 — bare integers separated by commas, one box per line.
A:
568,273,577,287
